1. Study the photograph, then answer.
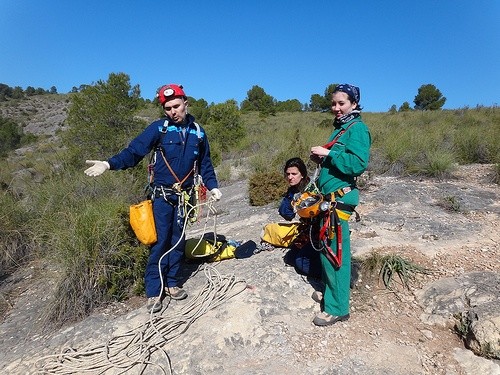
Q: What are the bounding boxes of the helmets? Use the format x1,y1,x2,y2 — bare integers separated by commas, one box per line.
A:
291,191,323,219
157,84,185,106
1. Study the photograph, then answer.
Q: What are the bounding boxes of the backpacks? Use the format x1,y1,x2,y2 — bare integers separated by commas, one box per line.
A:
184,223,304,262
147,119,201,183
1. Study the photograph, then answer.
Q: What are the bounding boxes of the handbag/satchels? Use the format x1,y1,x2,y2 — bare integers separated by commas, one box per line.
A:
130,200,158,246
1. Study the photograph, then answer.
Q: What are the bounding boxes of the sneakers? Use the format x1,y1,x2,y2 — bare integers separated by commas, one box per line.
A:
146,296,161,313
164,287,187,300
313,311,350,326
312,290,324,304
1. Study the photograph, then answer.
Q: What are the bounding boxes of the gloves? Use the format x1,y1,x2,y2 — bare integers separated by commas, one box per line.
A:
211,188,222,202
84,160,110,177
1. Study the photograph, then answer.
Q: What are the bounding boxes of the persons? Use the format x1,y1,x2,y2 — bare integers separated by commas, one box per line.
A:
278,157,353,289
310,84,371,326
84,83,223,313
